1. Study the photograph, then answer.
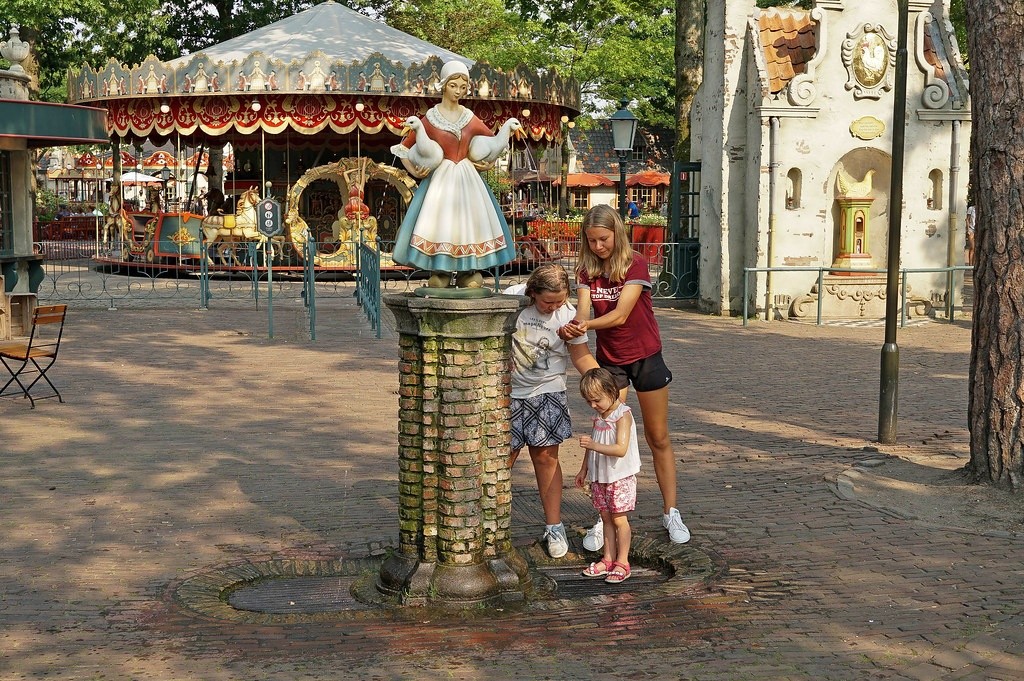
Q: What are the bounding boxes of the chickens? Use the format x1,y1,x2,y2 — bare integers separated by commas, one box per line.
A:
836,159,877,198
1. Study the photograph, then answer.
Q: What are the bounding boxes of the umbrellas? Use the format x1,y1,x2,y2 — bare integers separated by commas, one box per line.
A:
625,170,671,208
552,172,613,209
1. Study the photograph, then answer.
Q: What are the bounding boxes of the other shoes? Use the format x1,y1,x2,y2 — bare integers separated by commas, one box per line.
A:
543,521,570,559
663,508,691,544
583,514,604,551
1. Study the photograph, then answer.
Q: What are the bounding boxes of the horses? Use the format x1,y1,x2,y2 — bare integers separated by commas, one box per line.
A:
201,185,226,216
102,179,134,257
200,184,285,267
145,184,162,213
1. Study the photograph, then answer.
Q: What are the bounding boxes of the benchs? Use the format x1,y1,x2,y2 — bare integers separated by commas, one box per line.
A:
53,215,108,242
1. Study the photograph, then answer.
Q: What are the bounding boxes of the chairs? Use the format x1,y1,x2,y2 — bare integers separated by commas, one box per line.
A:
0,303,67,411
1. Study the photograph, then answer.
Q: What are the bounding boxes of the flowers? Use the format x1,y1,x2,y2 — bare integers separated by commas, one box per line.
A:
631,213,669,226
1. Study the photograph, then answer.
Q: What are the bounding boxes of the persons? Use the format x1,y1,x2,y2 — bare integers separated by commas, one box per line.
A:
575,368,641,583
392,60,516,287
509,264,600,558
501,190,544,217
625,196,645,219
556,205,690,551
92,189,165,211
196,190,208,217
966,205,975,271
38,206,104,238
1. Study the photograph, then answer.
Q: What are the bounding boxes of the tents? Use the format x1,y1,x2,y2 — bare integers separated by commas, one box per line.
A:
102,168,164,209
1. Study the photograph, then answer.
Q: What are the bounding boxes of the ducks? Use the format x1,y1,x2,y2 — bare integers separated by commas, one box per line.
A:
389,115,445,174
467,117,529,165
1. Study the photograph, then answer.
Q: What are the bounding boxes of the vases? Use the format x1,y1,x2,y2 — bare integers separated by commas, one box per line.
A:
633,224,668,265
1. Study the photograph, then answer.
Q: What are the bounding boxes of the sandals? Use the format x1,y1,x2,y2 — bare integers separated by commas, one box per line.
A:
582,558,615,577
604,561,631,583
